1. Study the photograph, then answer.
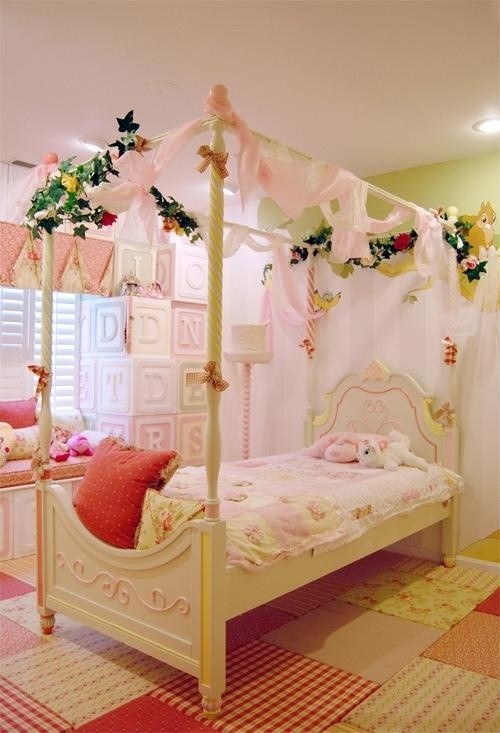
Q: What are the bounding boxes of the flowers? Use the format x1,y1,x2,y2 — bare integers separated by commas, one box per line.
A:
18,109,203,245
287,204,488,283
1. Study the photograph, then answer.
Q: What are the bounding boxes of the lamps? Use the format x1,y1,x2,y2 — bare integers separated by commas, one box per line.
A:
36,358,460,718
224,323,273,459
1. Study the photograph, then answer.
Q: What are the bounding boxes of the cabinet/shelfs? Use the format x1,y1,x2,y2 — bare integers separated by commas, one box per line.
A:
73,235,208,468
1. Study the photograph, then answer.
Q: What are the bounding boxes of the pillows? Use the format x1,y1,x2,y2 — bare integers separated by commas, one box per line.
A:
35,408,85,437
72,436,183,549
135,488,205,550
0,396,38,429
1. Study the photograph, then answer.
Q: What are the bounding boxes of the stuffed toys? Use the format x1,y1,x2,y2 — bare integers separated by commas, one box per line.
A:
309,432,387,464
357,433,428,473
0,422,94,467
